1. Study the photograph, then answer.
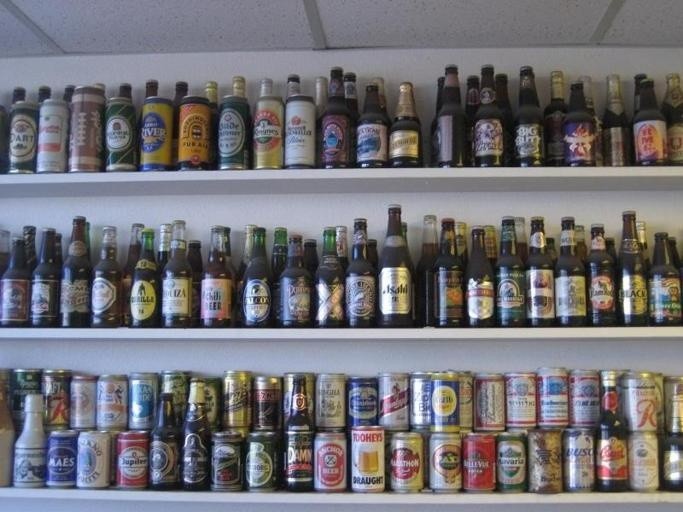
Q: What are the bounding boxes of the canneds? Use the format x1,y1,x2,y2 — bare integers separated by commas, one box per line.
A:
7,84,317,174
0,367,683,494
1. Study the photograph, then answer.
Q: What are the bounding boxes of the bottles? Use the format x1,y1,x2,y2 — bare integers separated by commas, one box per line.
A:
0,202,683,328
660,394,683,492
593,371,630,492
0,61,682,175
175,378,211,491
12,393,48,488
148,392,179,490
0,379,16,487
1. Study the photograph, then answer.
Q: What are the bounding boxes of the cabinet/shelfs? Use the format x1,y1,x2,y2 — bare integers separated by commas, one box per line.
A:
0,167,683,505
